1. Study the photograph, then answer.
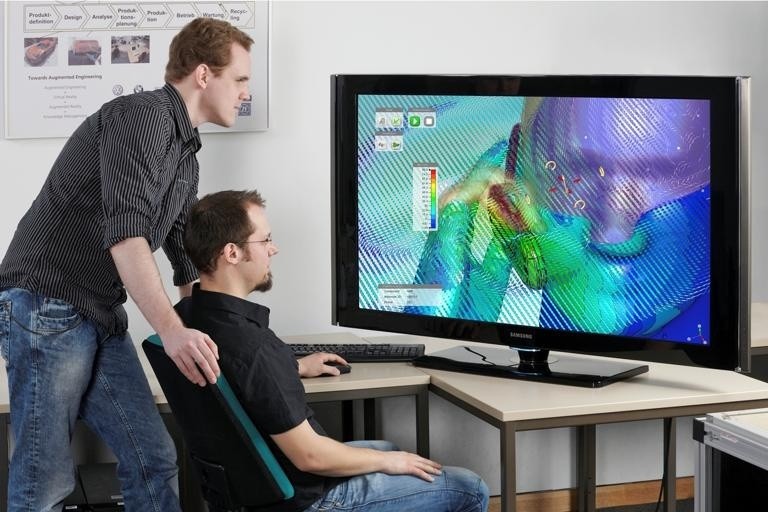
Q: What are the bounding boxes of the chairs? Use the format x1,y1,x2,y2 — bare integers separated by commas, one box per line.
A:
141,327,298,511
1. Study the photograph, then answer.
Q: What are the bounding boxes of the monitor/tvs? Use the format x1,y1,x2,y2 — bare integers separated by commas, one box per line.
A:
330,72,753,390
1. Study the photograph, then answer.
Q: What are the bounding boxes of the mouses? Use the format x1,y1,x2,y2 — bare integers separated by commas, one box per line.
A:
319,362,353,377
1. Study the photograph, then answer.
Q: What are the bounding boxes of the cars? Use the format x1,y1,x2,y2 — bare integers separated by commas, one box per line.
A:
24,39,56,66
125,41,149,63
73,40,99,57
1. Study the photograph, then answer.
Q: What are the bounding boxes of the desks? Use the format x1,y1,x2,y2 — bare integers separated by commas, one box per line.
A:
0,330,768,512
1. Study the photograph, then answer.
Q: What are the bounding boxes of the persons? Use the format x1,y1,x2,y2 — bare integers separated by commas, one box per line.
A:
174,188,489,511
0,16,257,512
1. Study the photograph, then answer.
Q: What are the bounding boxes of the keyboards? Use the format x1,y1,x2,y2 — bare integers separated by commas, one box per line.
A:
285,342,426,366
